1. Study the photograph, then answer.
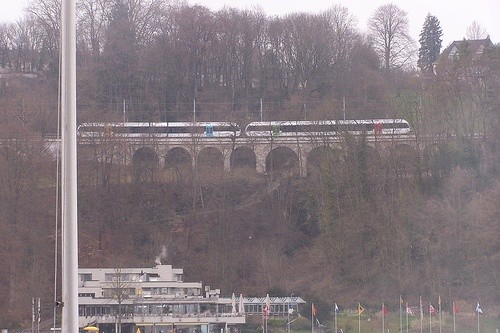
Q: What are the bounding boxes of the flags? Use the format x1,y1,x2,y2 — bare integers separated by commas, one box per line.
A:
359,306,366,313
407,307,413,315
430,305,436,315
312,305,317,316
476,303,482,313
265,304,270,314
453,305,459,314
382,305,388,317
334,304,344,313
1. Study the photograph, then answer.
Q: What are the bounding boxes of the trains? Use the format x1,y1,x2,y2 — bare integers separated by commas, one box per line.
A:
76,119,411,139
